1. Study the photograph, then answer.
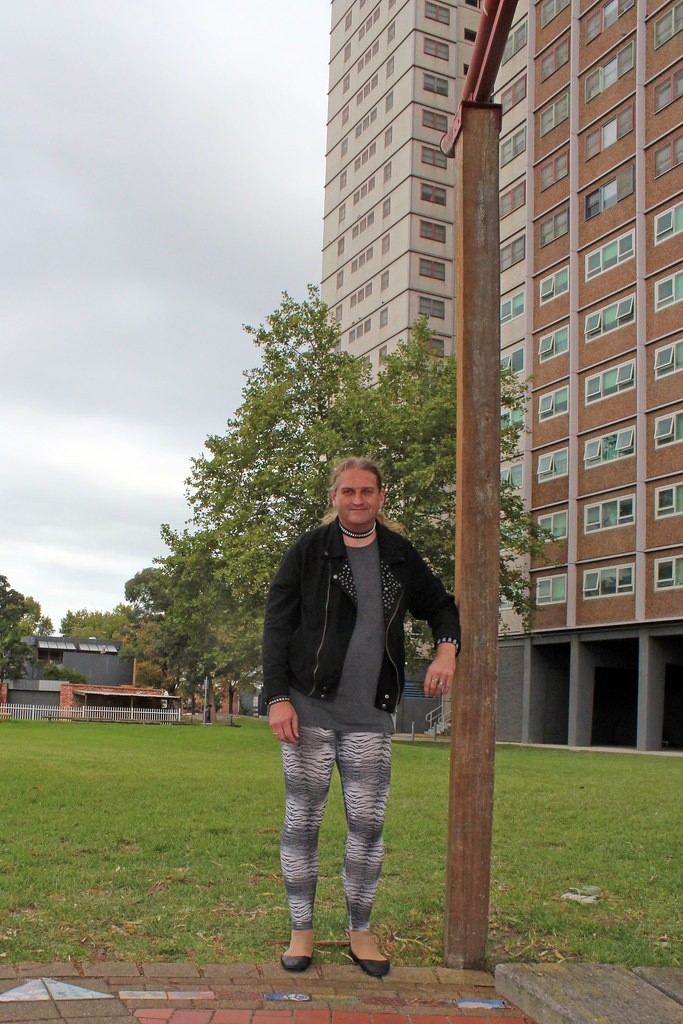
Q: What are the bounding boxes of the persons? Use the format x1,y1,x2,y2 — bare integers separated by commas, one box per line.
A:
262,457,462,978
204,704,212,723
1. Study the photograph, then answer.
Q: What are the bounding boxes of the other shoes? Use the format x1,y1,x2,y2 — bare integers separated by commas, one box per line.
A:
281,945,314,972
348,944,390,977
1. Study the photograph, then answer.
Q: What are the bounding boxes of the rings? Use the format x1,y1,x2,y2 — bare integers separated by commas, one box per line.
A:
432,678,439,684
438,681,444,685
273,732,278,736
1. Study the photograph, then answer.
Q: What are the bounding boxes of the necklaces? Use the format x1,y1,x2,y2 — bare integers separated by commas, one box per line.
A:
339,521,377,539
